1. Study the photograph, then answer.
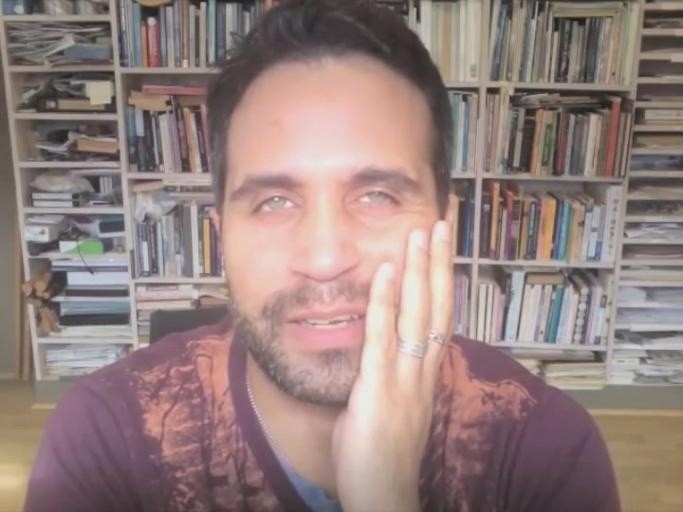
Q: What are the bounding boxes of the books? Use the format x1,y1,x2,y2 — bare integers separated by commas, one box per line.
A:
371,0,682,393
1,0,286,379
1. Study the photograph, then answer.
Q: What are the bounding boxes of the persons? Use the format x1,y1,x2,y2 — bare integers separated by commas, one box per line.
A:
21,0,623,510
